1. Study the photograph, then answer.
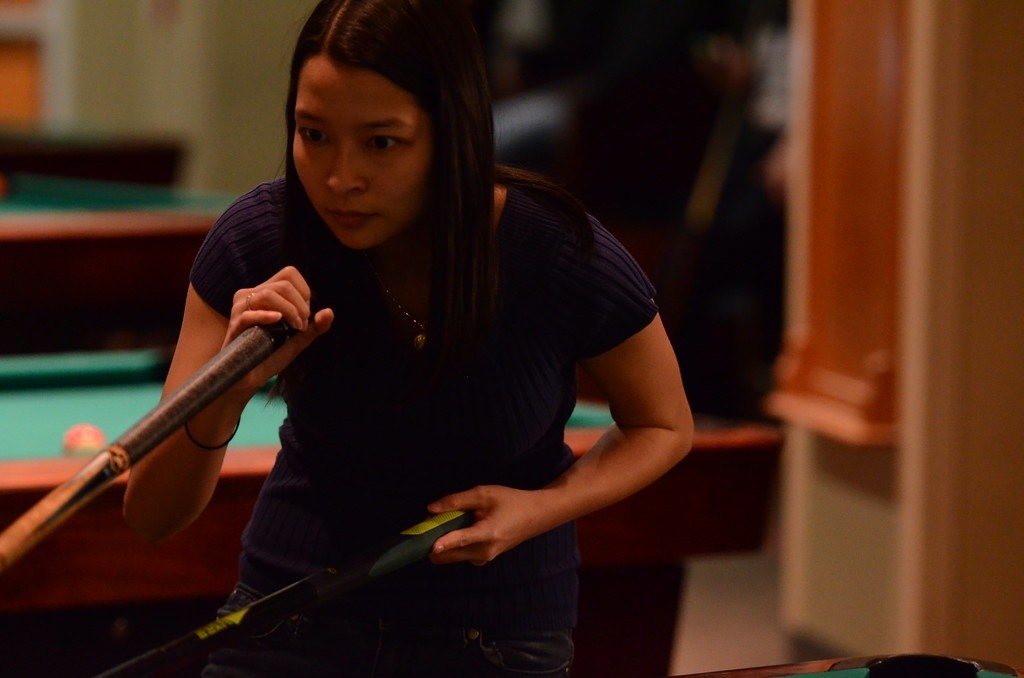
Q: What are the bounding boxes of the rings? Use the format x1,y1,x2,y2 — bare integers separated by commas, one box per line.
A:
246,292,256,310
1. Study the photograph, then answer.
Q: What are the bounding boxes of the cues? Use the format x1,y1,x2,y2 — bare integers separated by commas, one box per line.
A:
0,316,298,576
95,507,475,677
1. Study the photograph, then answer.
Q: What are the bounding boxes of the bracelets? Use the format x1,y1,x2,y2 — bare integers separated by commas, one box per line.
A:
185,416,241,450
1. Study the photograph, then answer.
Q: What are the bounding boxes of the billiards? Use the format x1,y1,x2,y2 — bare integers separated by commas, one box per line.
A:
63,424,106,460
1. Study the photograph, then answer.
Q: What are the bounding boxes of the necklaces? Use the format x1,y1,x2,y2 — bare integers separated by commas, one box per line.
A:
371,268,428,351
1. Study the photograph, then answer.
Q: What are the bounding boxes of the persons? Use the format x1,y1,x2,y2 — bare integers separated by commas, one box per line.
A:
122,0,694,678
